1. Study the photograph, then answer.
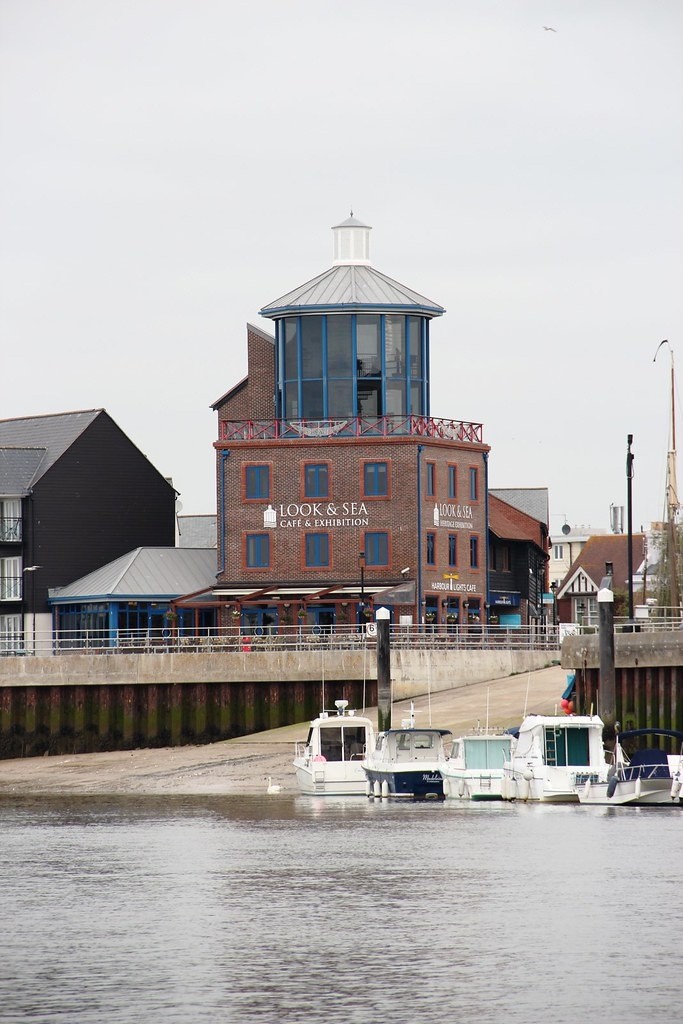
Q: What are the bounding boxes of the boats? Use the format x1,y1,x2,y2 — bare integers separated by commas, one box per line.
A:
501,713,613,802
572,721,683,806
292,700,378,796
438,726,513,802
360,728,453,801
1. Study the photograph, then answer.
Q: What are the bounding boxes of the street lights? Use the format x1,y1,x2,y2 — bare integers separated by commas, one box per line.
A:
538,562,545,634
20,566,38,656
549,582,558,634
653,339,676,623
357,551,367,623
579,604,586,635
